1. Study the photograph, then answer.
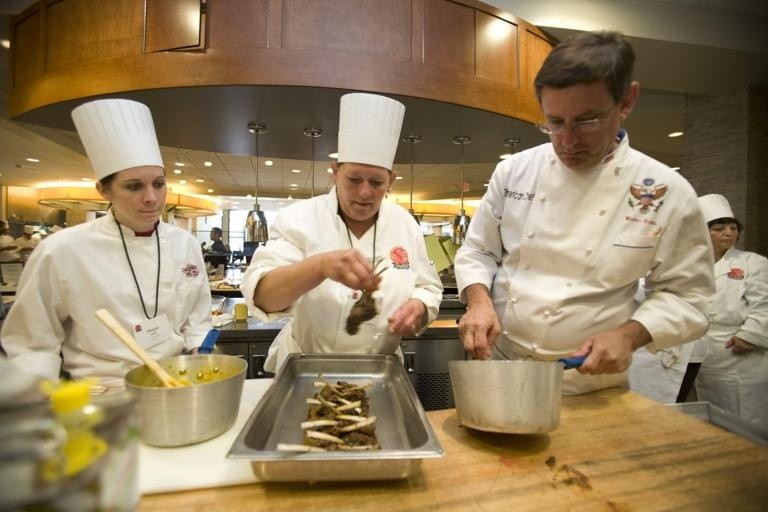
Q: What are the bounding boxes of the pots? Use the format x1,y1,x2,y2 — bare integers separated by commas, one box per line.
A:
125,329,248,448
447,355,588,435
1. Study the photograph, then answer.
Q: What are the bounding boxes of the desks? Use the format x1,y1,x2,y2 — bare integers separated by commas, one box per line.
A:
135,386,768,511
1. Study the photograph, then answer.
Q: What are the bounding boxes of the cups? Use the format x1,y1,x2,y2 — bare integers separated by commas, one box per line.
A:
234,304,248,320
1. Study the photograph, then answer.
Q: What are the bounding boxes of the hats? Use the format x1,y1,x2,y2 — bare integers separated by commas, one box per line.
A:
24,224,61,234
699,193,735,225
71,99,165,182
337,93,406,170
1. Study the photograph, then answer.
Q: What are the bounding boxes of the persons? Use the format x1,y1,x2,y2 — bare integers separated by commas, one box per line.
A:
630,267,707,410
695,216,767,402
456,30,717,396
242,242,259,265
202,228,228,272
239,160,444,377
1,166,214,400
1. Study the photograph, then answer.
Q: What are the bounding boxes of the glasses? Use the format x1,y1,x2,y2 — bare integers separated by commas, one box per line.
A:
534,102,620,134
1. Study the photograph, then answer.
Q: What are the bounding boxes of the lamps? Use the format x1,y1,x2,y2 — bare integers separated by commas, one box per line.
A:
403,135,422,225
453,136,470,244
245,125,269,243
